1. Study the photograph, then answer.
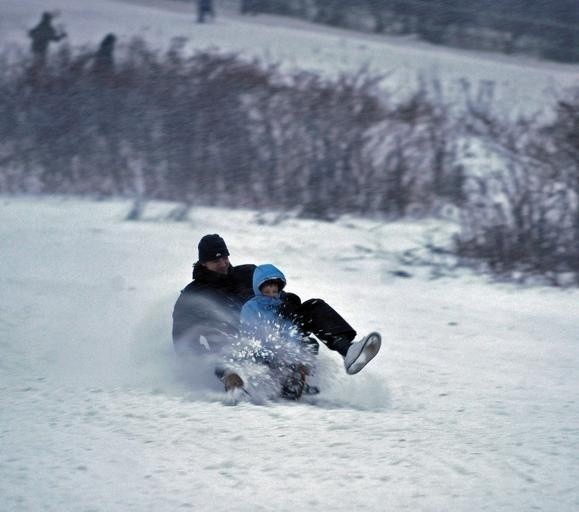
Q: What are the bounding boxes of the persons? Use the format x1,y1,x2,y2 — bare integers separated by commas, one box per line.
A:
29,12,69,59
171,233,382,399
195,0,215,23
93,31,118,70
238,263,321,404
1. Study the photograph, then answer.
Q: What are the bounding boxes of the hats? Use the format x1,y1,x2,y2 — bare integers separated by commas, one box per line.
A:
198,234,230,262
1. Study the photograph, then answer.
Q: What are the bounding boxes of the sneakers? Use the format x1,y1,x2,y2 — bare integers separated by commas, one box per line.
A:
342,331,382,375
